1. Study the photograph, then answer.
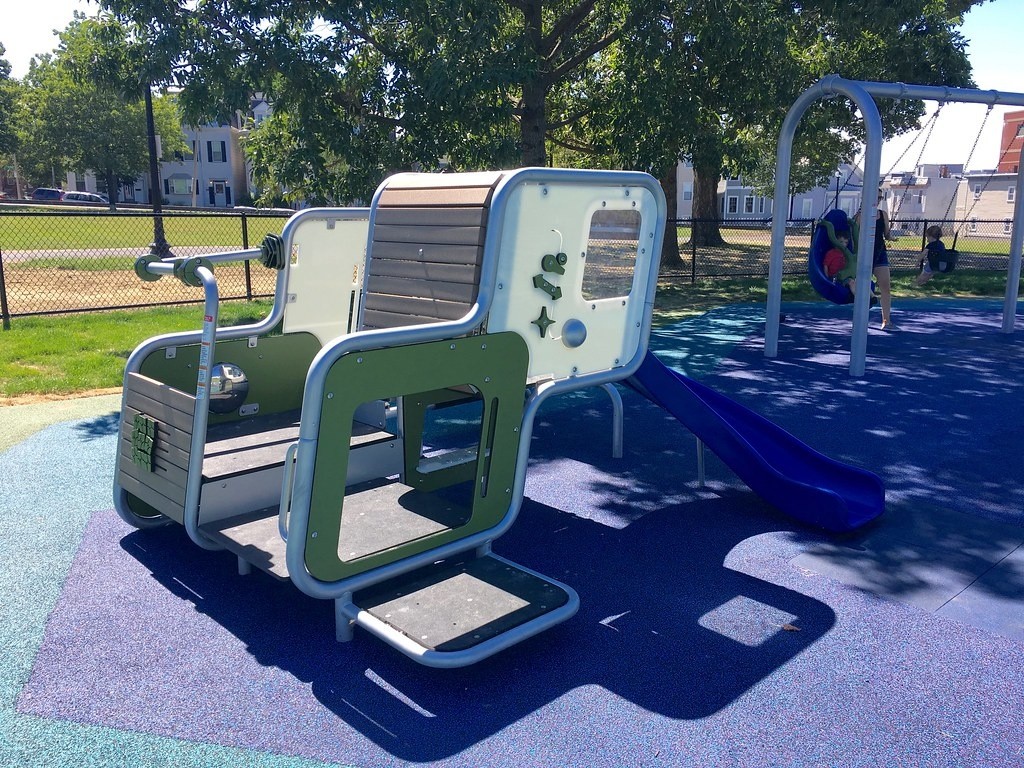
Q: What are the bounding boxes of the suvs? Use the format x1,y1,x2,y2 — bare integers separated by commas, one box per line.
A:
0,191,10,199
31,188,63,201
60,191,109,203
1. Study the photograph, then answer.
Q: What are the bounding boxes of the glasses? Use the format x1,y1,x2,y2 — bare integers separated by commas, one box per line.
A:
878,196,884,200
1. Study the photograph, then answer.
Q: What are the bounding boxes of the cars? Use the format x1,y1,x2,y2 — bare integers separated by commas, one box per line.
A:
766,214,812,235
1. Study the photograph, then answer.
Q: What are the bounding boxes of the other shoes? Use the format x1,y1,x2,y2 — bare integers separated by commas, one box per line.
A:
881,322,901,331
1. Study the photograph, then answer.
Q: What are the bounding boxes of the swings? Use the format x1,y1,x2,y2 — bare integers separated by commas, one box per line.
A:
927,103,1024,274
808,101,903,304
848,100,946,275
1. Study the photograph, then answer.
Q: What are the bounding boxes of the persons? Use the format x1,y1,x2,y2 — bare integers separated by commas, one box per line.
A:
856,189,902,332
909,226,945,289
823,231,877,309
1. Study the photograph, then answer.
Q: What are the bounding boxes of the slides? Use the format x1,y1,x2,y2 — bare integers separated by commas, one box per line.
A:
618,347,886,535
